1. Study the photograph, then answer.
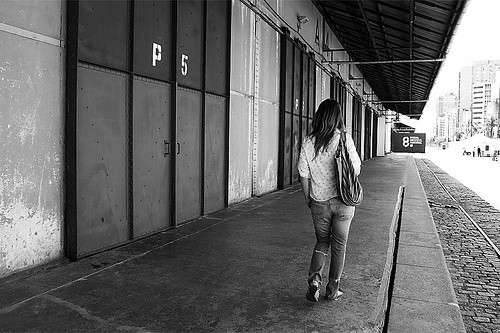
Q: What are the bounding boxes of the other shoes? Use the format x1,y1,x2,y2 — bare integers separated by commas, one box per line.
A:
334,288,345,301
305,281,320,302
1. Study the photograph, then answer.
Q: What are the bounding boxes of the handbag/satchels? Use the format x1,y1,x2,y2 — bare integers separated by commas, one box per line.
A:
334,131,363,206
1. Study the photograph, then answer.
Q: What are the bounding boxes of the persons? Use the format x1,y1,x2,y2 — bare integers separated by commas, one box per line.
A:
462,146,482,157
297,99,362,302
